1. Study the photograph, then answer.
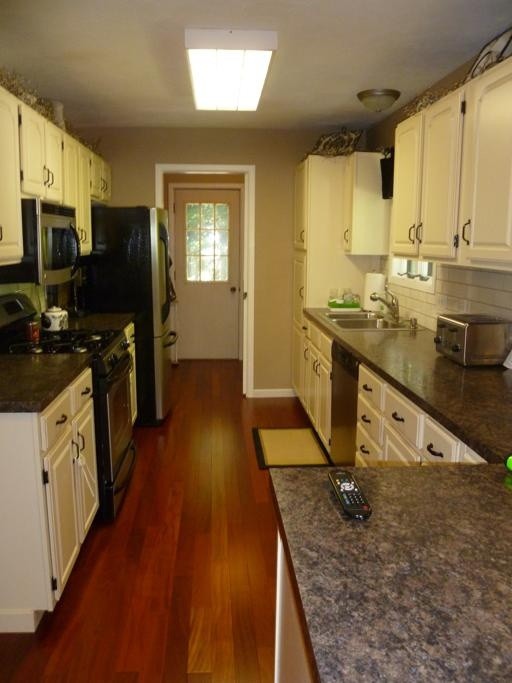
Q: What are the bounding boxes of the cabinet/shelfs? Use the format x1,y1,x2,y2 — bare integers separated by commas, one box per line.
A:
391,85,463,267
383,362,485,463
0,367,101,634
0,85,110,266
293,152,392,466
124,321,137,426
463,56,512,273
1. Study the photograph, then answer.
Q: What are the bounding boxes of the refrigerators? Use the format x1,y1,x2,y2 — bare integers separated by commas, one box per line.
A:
71,204,180,427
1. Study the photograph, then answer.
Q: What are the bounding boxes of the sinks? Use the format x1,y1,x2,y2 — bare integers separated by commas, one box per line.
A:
325,312,387,319
332,319,425,333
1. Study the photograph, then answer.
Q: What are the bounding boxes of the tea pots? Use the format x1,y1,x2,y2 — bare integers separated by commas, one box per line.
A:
41,304,69,332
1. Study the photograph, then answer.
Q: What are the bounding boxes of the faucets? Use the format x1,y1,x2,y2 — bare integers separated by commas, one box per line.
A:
370,285,400,322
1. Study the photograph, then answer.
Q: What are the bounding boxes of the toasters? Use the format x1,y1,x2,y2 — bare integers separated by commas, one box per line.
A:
433,312,512,369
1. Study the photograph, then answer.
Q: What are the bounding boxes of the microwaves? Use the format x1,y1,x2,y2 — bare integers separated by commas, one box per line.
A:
20,197,83,286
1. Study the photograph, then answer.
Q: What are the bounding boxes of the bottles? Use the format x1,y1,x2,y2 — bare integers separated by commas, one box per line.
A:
26,320,41,343
343,290,360,304
52,99,66,129
69,279,86,318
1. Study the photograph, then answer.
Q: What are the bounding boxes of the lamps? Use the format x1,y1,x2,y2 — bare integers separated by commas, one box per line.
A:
356,89,400,112
186,28,278,112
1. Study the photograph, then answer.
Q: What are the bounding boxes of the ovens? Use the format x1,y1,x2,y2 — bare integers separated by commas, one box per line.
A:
93,353,138,523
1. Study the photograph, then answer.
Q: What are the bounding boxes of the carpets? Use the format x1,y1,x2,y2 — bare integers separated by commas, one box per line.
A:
252,426,335,469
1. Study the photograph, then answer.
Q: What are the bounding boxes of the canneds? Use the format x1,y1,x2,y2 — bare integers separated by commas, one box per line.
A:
26,322,39,344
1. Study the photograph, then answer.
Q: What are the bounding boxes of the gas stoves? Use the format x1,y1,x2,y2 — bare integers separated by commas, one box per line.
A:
1,327,131,377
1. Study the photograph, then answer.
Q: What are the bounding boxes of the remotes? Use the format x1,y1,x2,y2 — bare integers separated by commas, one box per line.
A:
328,469,372,520
